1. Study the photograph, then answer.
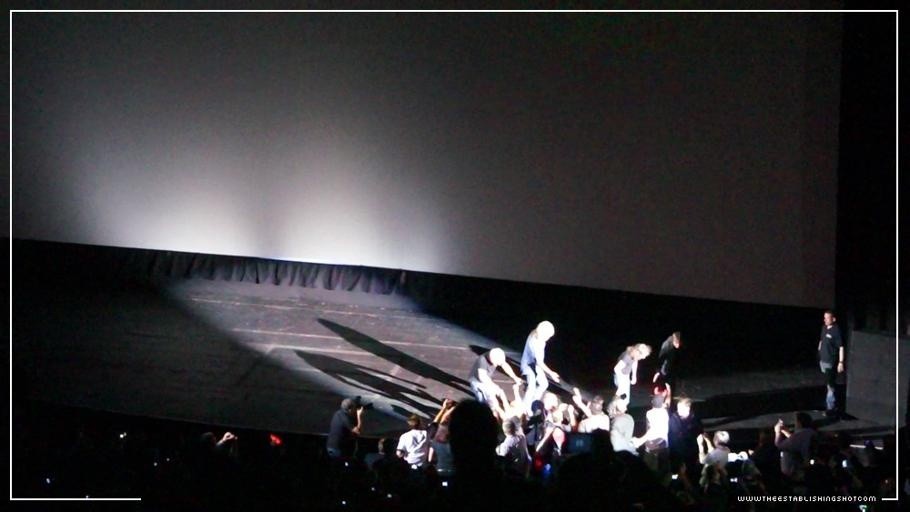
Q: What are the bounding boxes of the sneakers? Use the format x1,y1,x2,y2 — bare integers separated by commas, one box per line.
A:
822,408,839,416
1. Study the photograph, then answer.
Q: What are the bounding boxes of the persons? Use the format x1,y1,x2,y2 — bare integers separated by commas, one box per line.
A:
188,309,910,512
